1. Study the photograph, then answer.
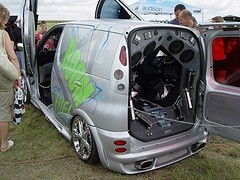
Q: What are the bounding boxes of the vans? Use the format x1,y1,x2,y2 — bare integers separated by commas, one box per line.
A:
15,0,240,175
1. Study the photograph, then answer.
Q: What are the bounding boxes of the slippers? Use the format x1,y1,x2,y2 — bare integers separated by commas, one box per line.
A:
1,141,14,152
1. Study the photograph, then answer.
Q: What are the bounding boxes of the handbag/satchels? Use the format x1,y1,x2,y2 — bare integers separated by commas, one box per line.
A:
0,31,20,80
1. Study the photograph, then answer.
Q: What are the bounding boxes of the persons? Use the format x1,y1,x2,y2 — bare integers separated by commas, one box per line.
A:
178,9,199,28
211,16,227,29
0,3,55,153
170,4,197,25
